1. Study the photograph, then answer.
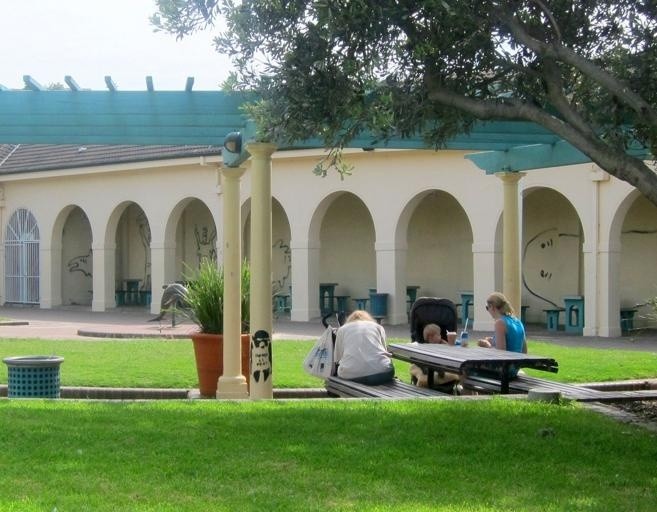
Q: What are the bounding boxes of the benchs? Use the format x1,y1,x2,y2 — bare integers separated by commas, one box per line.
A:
542,308,580,330
351,296,416,315
462,374,599,393
116,290,125,305
454,303,474,324
521,305,530,323
320,295,351,314
275,295,292,313
140,290,151,305
324,375,455,398
619,310,637,332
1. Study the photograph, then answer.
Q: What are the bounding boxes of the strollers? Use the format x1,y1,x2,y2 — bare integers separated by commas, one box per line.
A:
321,310,347,345
410,296,458,391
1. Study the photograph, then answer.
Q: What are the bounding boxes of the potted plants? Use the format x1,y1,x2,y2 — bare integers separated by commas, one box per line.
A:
163,254,249,395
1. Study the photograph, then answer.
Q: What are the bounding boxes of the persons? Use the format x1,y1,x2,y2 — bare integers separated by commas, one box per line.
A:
462,292,528,395
408,324,459,387
333,309,394,386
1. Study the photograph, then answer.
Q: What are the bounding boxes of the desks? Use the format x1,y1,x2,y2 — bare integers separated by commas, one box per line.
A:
284,284,337,314
121,279,142,304
456,291,475,327
563,296,586,330
387,343,558,394
367,286,421,310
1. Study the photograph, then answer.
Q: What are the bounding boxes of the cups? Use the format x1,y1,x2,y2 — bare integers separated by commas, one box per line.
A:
447,332,457,345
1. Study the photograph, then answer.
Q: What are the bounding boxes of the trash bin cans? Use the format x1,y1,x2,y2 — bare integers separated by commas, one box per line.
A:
3,354,67,400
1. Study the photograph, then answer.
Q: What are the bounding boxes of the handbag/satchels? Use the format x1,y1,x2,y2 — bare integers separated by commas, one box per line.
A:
303,324,335,379
